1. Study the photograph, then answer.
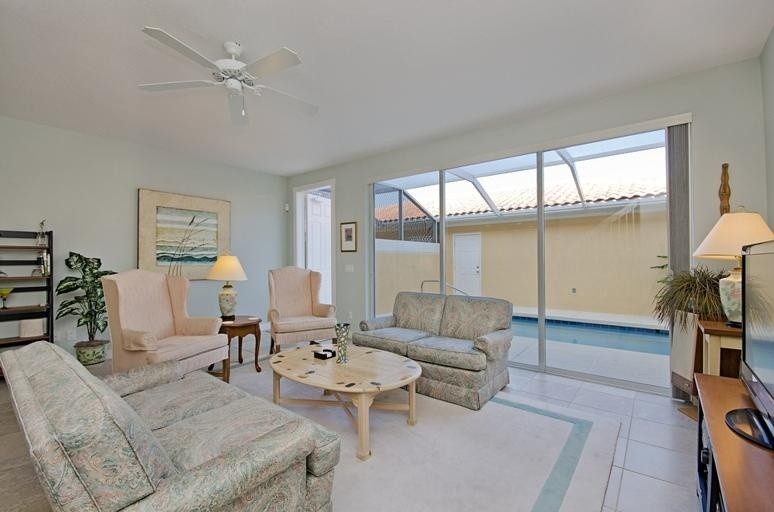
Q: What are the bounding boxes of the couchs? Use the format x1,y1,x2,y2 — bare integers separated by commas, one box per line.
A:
101,267,230,391
2,339,341,512
265,264,338,357
352,291,514,414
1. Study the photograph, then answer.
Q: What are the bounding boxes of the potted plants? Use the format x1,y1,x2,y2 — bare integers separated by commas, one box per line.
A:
55,251,119,368
654,265,729,323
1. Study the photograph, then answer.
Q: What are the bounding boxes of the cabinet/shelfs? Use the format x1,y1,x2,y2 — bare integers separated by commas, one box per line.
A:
0,229,54,347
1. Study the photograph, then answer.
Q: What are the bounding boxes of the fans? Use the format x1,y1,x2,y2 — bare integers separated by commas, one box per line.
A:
135,23,321,130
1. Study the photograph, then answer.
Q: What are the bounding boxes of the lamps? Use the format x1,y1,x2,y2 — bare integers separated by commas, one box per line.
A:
692,209,774,329
205,255,248,324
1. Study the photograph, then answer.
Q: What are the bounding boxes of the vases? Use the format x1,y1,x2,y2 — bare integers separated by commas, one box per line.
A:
333,323,351,364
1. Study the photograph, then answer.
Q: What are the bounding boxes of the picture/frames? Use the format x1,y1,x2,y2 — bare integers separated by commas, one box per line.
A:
340,222,358,253
136,187,238,283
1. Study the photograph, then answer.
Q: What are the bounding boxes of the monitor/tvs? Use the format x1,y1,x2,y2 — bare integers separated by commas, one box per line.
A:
726,240,774,450
